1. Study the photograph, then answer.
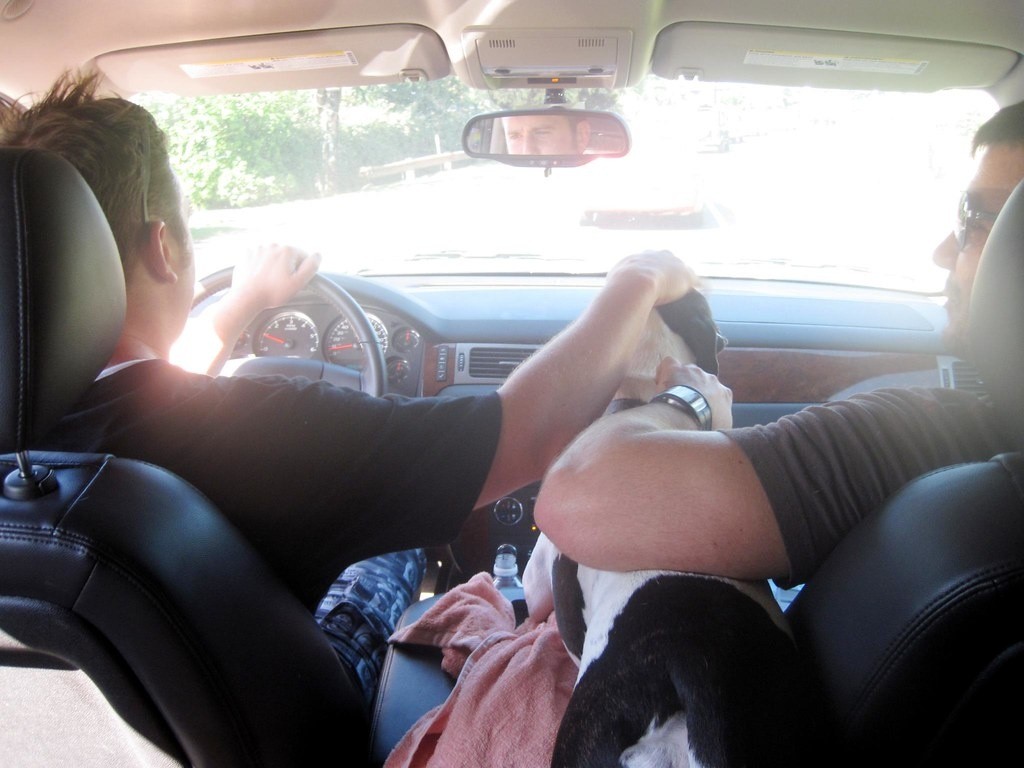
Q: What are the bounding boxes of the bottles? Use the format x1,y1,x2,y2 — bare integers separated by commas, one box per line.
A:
491,543,524,590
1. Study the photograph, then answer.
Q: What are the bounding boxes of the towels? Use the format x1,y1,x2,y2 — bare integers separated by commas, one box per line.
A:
380,571,579,768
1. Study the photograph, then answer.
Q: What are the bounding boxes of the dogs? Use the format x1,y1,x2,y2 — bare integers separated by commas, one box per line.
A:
521,284,826,768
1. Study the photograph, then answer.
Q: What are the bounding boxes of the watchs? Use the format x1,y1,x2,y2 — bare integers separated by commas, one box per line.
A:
649,385,713,431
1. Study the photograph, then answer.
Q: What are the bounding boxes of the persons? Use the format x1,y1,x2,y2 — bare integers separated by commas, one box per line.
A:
502,116,591,155
1,66,697,718
532,105,1024,591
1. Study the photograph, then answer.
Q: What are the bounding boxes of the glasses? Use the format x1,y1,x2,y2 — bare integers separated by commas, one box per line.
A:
954,191,999,251
96,98,156,227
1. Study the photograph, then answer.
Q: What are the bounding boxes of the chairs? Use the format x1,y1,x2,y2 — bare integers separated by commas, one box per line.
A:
0,147,371,768
778,176,1024,768
